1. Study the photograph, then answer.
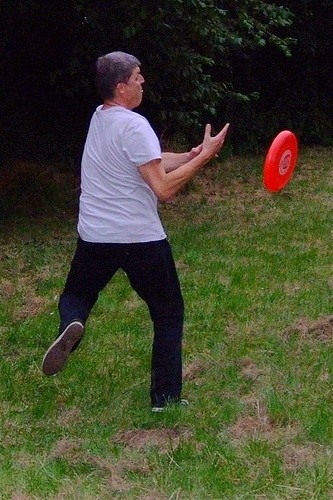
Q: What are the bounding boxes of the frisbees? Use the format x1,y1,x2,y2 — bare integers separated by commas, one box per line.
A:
262,130,299,192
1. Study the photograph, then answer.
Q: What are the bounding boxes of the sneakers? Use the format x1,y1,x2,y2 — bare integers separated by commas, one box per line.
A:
151,398,189,413
41,319,85,375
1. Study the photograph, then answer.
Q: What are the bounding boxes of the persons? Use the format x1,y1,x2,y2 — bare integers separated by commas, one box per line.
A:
42,51,230,412
